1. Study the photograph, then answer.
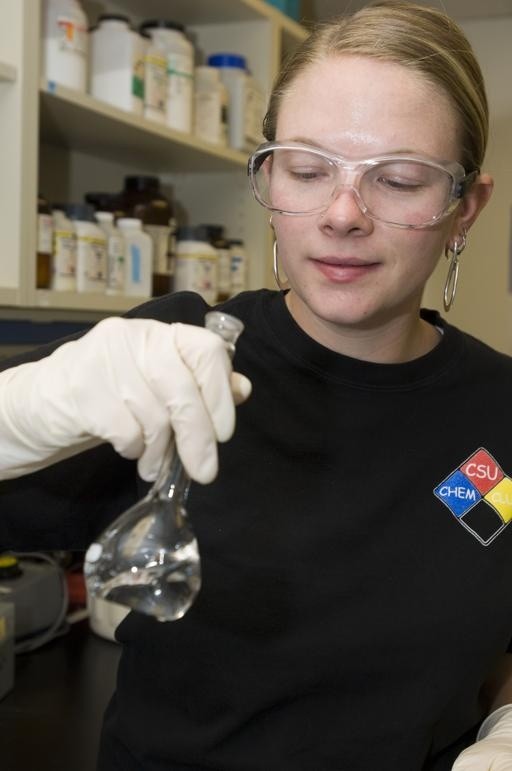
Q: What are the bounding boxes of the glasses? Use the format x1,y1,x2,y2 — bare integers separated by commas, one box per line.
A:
247,139,481,232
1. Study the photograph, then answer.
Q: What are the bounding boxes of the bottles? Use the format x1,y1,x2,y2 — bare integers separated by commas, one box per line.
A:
85,310,243,640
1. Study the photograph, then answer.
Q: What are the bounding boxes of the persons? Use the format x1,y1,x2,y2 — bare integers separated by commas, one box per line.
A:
0,0,512,771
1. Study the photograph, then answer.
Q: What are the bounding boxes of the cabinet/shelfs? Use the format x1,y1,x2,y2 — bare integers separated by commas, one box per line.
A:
2,0,311,323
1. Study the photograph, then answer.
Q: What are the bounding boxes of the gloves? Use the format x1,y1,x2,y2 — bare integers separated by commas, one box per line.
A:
1,315,254,486
450,703,511,771
